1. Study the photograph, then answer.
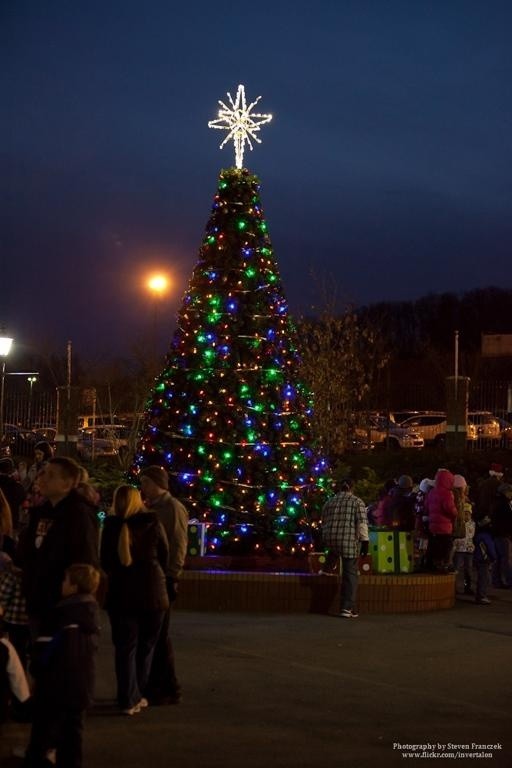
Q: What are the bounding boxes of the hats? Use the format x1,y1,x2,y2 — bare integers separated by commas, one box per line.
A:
478,515,492,528
142,466,170,490
384,469,468,494
463,504,472,513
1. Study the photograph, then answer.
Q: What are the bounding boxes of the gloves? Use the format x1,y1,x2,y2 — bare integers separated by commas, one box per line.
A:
164,576,178,601
360,541,368,558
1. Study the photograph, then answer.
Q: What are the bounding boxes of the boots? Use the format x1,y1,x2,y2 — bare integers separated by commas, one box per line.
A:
463,582,475,596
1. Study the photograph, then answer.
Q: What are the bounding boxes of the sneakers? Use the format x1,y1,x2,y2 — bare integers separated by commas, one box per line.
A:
479,598,491,604
321,568,335,577
339,606,360,618
447,566,460,574
115,689,182,716
496,583,512,590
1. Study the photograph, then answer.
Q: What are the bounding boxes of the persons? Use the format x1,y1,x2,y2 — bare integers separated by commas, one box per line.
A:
0,418,112,767
367,456,512,605
319,477,371,619
98,483,172,715
137,464,191,706
